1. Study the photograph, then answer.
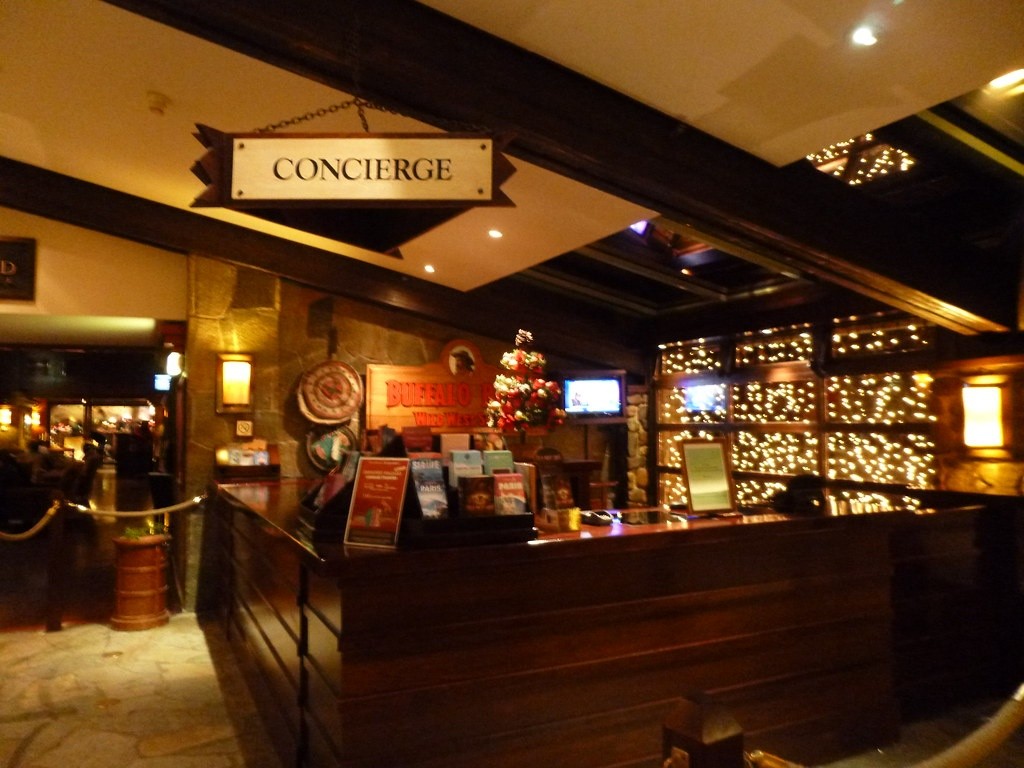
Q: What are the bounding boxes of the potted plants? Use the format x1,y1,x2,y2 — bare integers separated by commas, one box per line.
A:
108,521,173,630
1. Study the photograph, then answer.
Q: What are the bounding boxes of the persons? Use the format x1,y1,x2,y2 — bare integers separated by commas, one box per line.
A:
80,442,103,492
15,438,46,484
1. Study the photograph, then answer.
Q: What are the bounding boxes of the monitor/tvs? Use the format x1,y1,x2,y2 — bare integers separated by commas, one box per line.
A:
684,383,728,411
565,374,622,418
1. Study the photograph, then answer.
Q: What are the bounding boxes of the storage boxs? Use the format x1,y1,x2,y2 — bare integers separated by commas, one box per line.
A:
447,449,484,488
495,467,528,515
455,474,495,515
293,434,401,539
484,447,515,475
399,436,541,548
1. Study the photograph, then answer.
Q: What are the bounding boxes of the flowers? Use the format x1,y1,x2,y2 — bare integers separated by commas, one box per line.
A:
484,328,566,436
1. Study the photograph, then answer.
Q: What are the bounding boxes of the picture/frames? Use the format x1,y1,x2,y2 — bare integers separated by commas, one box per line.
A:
680,438,741,513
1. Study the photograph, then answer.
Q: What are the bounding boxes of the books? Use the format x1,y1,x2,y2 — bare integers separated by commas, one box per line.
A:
411,446,528,519
